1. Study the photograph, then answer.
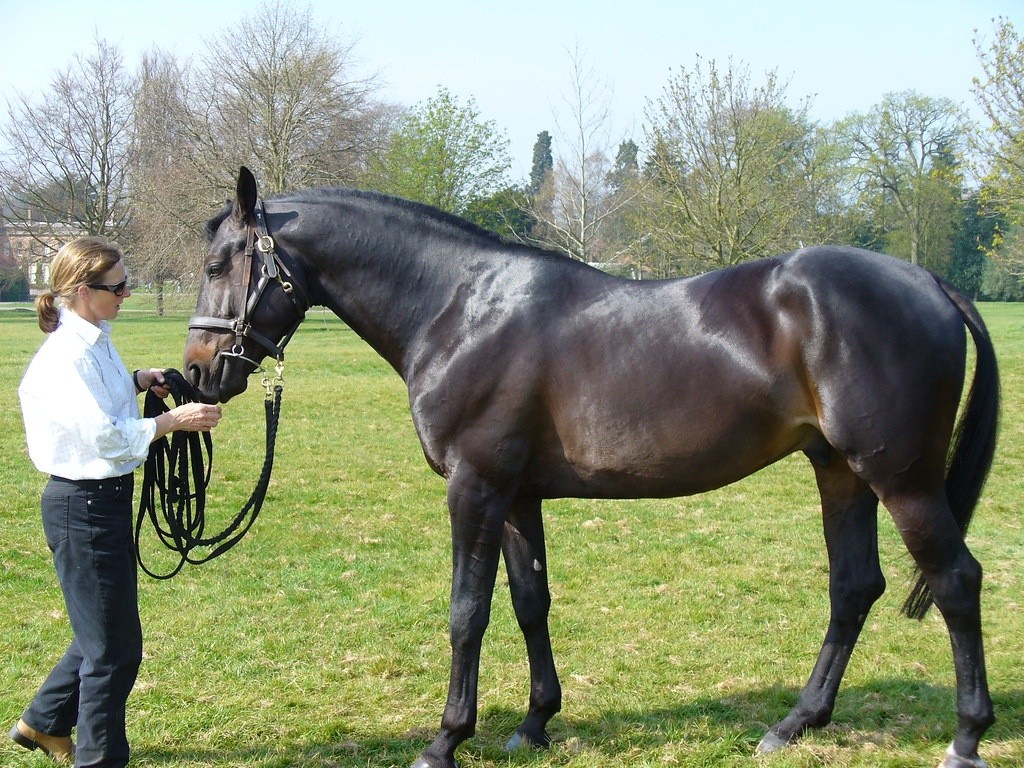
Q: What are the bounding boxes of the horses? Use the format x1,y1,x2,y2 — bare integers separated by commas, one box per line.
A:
178,160,1004,768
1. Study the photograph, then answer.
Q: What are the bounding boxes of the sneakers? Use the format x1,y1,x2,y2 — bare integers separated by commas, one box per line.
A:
9,719,75,764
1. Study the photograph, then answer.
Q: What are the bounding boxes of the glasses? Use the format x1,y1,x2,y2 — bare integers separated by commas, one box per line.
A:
86,275,127,296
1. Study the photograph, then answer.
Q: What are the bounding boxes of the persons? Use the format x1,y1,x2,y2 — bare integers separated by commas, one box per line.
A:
9,235,223,768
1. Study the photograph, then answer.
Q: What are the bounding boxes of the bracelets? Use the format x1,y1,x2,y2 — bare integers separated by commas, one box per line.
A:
133,370,147,393
